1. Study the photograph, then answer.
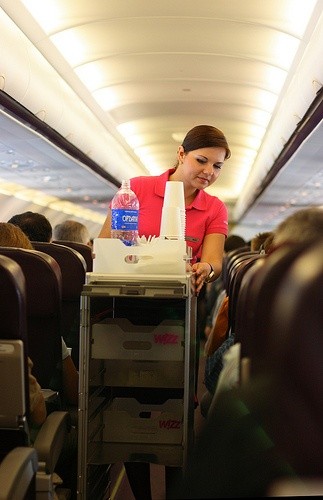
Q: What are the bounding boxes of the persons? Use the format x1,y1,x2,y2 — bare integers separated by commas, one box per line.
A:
90,123,229,500
0,210,94,433
204,208,323,455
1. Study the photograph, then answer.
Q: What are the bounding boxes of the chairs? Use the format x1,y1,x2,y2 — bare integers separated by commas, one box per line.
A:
0,240,323,500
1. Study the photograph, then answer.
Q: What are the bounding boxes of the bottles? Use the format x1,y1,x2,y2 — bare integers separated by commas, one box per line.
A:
159,181,186,241
111,179,140,264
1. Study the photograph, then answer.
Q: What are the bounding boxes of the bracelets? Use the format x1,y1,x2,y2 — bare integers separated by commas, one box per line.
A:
207,263,216,281
